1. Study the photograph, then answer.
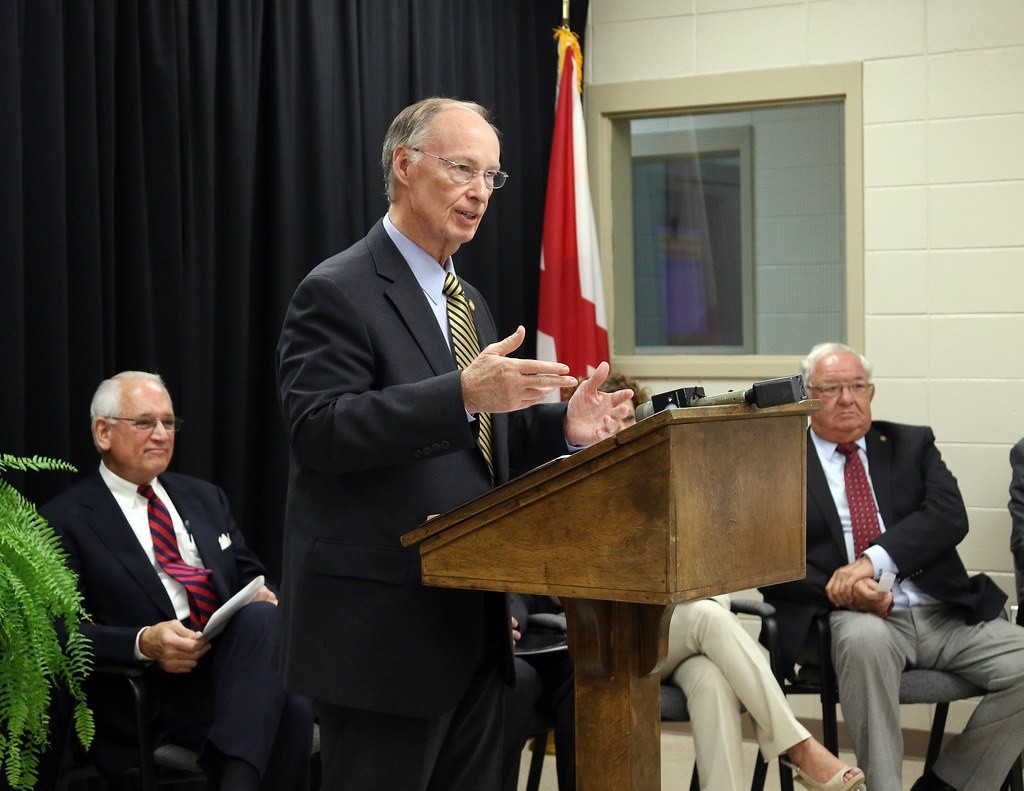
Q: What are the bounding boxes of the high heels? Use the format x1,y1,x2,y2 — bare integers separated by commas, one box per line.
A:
781,750,865,791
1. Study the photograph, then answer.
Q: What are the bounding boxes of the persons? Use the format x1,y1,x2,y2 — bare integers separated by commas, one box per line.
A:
39,372,315,791
258,97,634,791
756,342,1024,791
570,372,867,791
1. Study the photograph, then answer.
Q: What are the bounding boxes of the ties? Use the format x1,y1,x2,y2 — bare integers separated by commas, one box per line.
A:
137,482,220,631
443,272,496,477
836,441,882,563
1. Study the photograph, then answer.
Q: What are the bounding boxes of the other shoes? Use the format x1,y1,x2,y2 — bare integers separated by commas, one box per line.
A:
908,777,955,791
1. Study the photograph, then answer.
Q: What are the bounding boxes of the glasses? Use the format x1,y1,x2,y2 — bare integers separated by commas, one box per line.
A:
808,377,870,397
104,412,178,432
407,145,509,189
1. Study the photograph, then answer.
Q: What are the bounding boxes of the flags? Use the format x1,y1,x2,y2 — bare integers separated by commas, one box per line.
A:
536,26,611,399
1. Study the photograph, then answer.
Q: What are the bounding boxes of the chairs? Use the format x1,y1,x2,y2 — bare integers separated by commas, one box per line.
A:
51,663,322,791
748,601,1023,791
522,597,781,791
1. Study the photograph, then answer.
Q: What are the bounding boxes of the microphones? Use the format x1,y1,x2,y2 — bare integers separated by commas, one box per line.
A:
185,519,191,533
635,376,808,422
